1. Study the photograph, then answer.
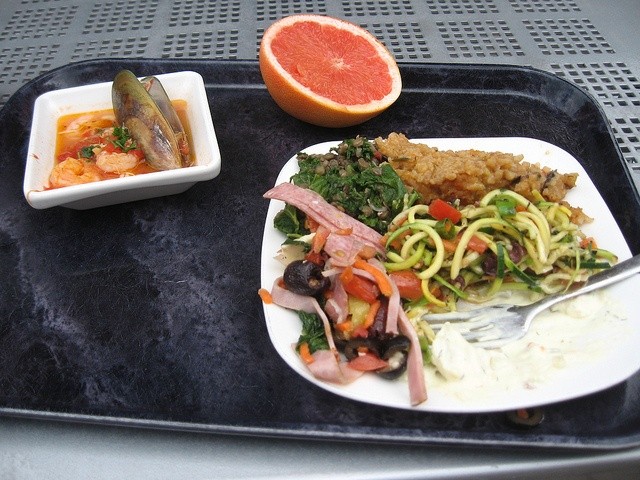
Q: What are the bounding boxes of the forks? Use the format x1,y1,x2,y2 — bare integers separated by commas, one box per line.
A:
425,252,636,350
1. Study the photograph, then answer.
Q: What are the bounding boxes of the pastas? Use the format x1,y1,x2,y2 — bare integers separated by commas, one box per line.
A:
381,195,617,308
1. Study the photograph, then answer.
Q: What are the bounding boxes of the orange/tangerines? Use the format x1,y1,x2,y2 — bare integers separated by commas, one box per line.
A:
258,14,404,129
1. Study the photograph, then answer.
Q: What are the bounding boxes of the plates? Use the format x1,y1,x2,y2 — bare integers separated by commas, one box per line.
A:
258,136,636,414
23,69,222,214
